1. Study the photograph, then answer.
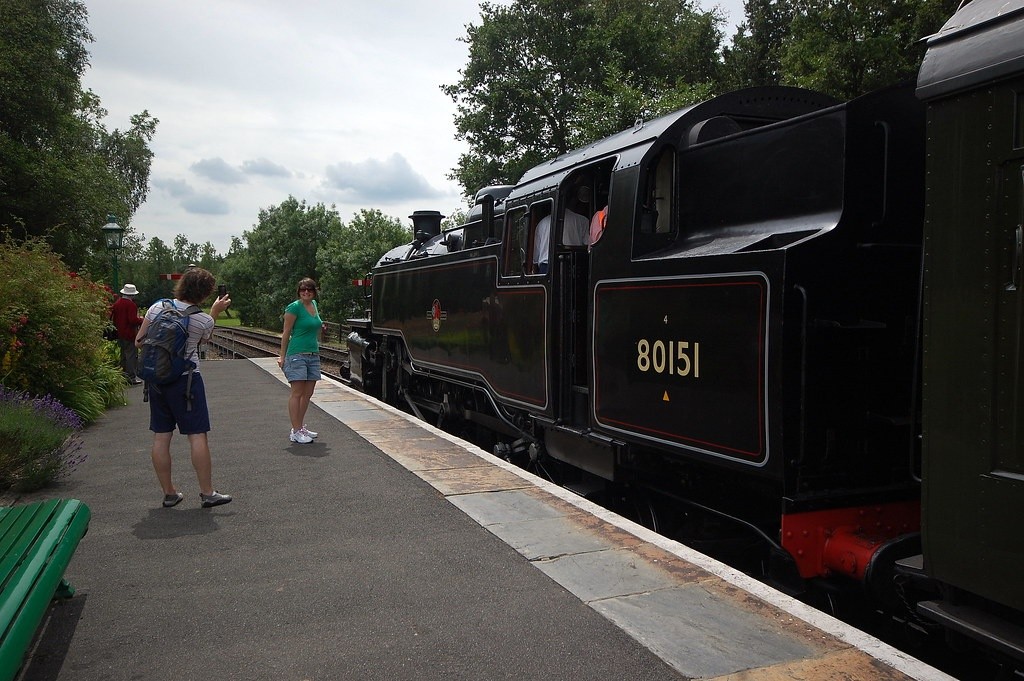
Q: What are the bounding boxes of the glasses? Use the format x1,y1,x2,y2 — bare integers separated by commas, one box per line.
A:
299,286,315,292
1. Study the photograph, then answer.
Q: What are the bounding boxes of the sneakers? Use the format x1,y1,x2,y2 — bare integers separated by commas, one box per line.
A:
301,427,318,438
162,492,183,507
199,490,232,507
290,427,313,444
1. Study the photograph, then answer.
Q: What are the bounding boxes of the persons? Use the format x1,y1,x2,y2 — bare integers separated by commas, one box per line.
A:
533,208,588,274
112,283,144,384
135,267,232,509
277,277,323,443
589,205,609,244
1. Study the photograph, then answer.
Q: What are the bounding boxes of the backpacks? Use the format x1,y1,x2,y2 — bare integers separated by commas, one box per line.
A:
135,296,204,386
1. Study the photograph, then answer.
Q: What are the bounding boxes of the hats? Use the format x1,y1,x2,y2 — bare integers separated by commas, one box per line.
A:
120,284,139,295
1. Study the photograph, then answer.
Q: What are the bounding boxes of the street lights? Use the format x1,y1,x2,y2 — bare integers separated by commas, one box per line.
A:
101,211,125,295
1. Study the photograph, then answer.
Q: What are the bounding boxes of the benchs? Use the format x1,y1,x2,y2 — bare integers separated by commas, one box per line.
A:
0,499,91,681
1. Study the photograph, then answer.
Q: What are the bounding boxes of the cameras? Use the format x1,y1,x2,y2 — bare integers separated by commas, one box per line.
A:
217,283,226,300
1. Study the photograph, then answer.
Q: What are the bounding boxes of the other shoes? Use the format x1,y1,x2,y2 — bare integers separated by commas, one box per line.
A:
126,379,142,386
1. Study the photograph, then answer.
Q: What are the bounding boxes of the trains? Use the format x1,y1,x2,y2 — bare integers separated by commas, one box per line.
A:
338,1,1023,681
